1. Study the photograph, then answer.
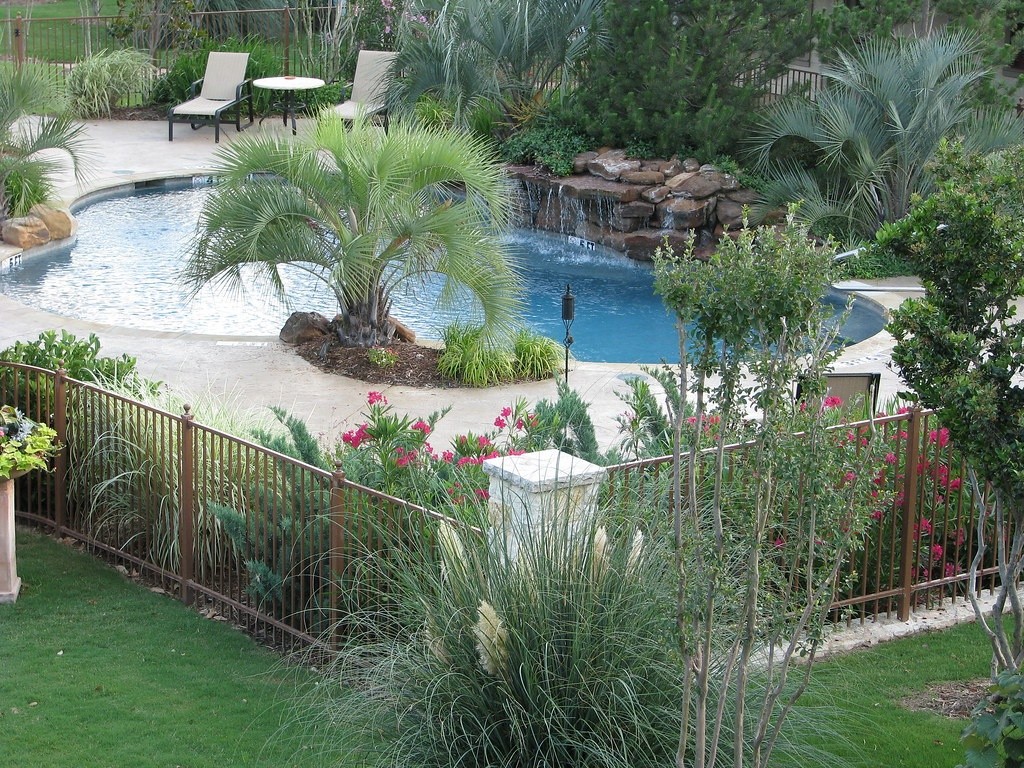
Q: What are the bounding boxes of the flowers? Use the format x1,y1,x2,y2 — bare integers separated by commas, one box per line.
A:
0,405,67,479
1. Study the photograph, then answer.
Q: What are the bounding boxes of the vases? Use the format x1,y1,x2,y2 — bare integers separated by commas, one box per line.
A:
0,460,32,481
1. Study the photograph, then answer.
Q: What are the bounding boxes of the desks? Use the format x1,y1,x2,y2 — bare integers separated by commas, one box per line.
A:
252,77,325,135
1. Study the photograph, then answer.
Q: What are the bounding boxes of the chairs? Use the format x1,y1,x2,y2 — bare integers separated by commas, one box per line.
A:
169,52,254,143
327,50,400,136
